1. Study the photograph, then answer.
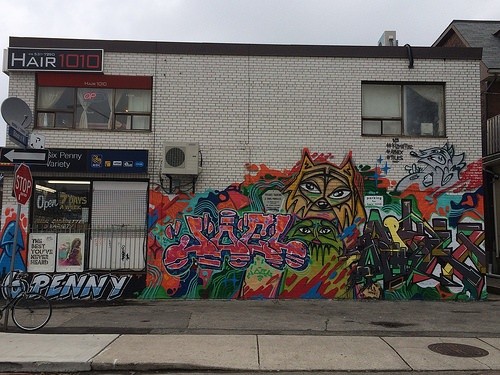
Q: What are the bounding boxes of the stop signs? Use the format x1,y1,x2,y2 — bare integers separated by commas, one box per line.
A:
13,163,33,205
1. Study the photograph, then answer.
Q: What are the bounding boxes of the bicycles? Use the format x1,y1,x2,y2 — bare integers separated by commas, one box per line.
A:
0,270,52,331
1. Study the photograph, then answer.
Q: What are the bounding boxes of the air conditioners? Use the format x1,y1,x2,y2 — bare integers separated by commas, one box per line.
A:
162,141,199,176
377,31,397,47
